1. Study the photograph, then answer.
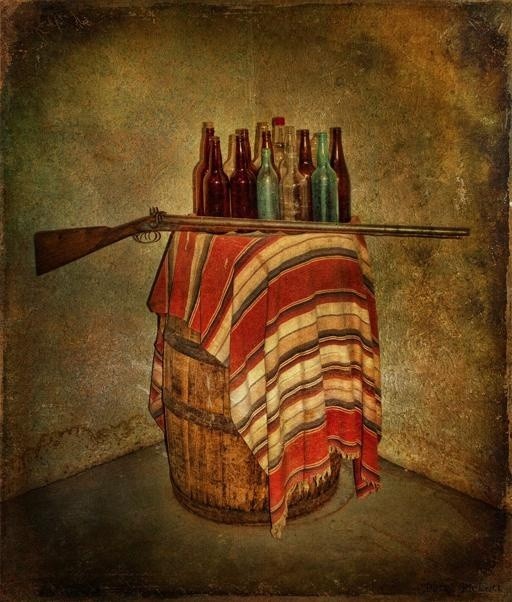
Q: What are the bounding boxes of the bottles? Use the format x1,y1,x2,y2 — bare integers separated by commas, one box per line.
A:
191,116,351,224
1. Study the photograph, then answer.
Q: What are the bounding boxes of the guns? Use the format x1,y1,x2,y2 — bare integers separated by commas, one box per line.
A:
33,208,470,278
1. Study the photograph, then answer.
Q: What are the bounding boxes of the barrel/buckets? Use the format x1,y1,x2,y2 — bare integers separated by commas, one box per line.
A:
162,231,339,526
